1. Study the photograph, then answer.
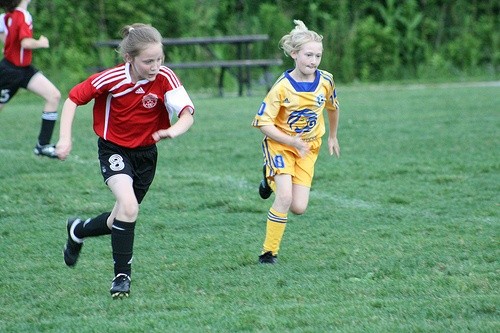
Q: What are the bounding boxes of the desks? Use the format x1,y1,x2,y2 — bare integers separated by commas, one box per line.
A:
97,34,268,97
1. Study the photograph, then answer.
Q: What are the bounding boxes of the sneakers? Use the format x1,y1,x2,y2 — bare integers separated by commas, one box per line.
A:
34,139,59,157
259,250,278,263
62,217,86,268
110,273,132,297
259,163,272,199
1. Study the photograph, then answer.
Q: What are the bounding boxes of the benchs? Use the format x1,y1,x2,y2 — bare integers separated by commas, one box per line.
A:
163,58,284,92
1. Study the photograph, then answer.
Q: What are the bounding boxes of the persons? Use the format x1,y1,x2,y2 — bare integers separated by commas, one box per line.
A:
55,21,195,300
250,19,340,264
0,0,62,159
